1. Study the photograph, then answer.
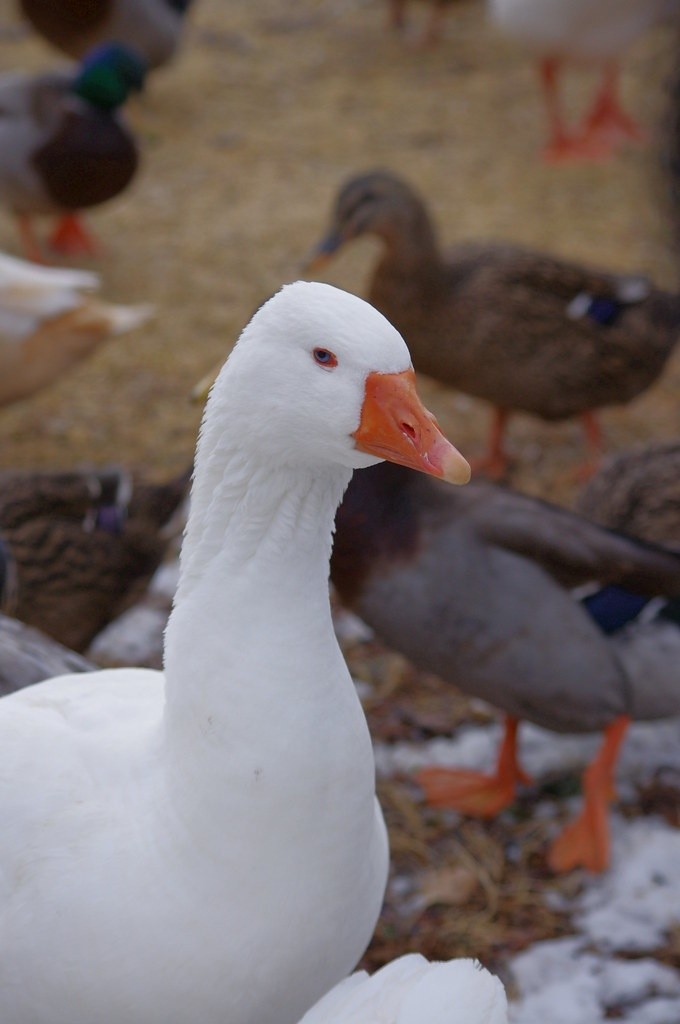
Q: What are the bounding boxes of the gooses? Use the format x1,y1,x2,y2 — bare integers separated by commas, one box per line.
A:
1,165,678,1022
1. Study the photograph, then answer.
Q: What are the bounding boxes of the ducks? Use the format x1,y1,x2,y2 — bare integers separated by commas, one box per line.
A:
488,1,658,164
386,0,472,49
0,1,196,414
